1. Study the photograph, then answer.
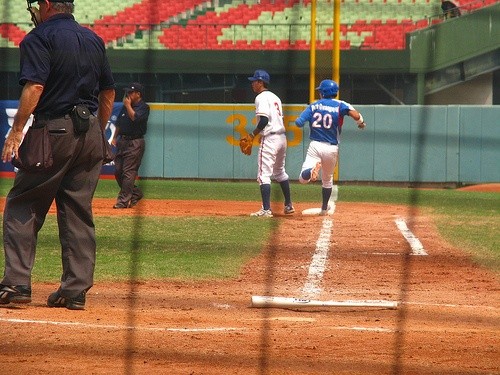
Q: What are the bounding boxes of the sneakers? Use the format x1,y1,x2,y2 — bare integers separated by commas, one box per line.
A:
129,192,143,208
250,205,273,217
311,162,321,181
0,284,32,304
113,204,127,208
321,206,330,216
284,203,295,214
47,290,85,310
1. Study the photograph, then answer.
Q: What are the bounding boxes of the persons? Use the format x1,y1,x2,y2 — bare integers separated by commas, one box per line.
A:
0,0,117,311
110,82,149,209
296,80,366,216
239,69,295,217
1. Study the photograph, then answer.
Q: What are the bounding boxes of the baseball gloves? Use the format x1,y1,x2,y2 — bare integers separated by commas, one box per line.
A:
239,135,253,156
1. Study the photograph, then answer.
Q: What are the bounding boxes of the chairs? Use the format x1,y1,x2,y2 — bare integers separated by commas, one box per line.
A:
0,0,500,51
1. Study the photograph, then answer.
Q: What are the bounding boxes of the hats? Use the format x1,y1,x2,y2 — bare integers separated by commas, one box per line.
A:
316,79,338,97
123,82,144,96
248,69,269,83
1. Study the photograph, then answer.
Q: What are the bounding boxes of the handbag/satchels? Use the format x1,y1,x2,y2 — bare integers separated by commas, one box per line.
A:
11,124,55,174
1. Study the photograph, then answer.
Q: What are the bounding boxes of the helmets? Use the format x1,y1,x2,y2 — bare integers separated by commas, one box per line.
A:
27,0,74,27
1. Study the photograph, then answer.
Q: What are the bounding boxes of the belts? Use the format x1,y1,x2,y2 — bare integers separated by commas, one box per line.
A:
120,134,141,140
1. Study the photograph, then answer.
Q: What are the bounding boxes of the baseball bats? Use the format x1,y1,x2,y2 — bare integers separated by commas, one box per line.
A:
251,295,399,310
